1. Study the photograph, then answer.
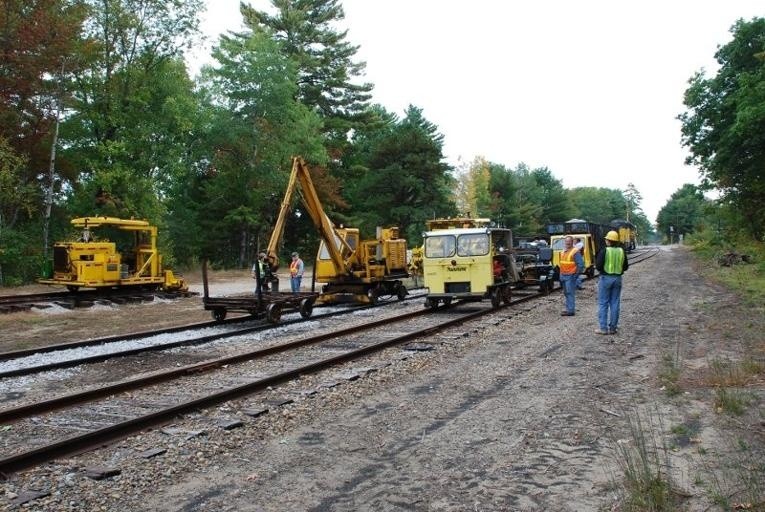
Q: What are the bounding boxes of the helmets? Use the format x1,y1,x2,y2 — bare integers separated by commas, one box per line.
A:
602,230,621,242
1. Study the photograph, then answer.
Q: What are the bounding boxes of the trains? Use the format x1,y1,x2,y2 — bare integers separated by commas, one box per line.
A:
546,218,635,253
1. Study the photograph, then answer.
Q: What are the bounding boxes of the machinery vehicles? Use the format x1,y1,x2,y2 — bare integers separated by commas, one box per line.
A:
264,154,413,306
36,214,189,293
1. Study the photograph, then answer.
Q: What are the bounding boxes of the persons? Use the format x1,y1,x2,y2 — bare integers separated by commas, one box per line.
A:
251,253,270,293
573,238,586,290
593,230,629,335
289,251,305,292
558,235,585,316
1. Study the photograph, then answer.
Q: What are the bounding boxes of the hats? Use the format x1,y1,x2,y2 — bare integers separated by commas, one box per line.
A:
291,251,298,257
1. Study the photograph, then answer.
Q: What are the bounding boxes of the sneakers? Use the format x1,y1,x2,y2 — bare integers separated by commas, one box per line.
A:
594,328,617,335
560,310,575,316
